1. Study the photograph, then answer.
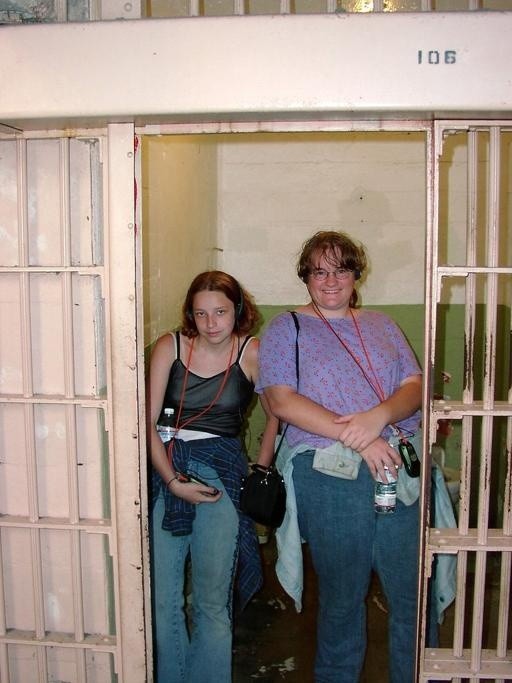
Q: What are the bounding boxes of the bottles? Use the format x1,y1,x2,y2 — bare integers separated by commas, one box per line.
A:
174,471,221,498
156,408,176,444
374,442,397,515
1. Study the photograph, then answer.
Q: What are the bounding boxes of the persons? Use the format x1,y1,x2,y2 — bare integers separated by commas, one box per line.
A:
251,230,438,683
147,269,280,683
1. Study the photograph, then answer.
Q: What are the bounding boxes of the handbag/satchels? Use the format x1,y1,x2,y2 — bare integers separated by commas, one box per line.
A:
241,465,285,527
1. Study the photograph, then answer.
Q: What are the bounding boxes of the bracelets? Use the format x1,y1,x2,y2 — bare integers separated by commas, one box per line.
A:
167,477,177,486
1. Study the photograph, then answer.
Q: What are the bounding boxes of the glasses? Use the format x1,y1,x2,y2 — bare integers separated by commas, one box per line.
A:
311,268,357,280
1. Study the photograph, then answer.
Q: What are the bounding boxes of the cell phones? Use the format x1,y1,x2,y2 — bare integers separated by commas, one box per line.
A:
187,474,218,495
398,438,420,477
